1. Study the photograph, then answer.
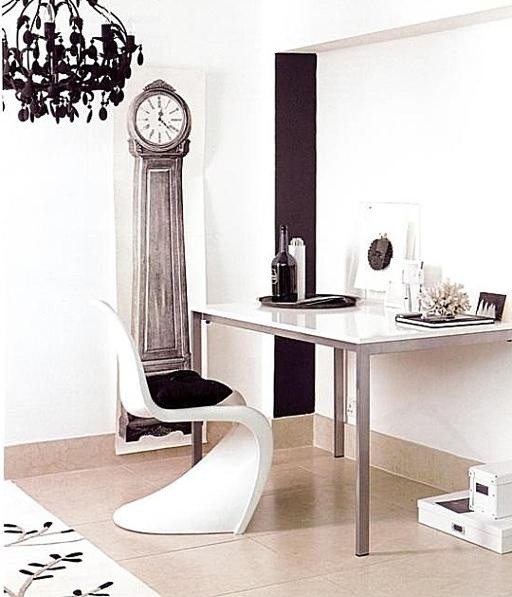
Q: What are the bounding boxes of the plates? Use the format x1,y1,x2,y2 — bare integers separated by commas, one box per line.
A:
256,292,360,309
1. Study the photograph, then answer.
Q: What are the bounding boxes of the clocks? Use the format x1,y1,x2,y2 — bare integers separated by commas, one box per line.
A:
125,74,193,376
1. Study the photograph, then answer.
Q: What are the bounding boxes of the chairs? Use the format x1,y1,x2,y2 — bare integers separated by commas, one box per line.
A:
94,297,275,536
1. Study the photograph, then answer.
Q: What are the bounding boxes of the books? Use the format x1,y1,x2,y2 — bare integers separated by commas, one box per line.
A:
396,311,495,329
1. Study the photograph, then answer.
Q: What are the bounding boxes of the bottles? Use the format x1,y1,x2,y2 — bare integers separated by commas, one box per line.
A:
271,224,306,302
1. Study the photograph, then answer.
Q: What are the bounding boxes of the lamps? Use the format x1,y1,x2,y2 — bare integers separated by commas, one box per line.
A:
0,0,144,128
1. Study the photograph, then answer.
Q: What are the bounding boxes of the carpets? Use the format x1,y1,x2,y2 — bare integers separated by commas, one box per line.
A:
4,475,161,597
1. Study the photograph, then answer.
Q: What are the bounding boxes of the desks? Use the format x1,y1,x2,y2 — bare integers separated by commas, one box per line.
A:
187,303,512,555
